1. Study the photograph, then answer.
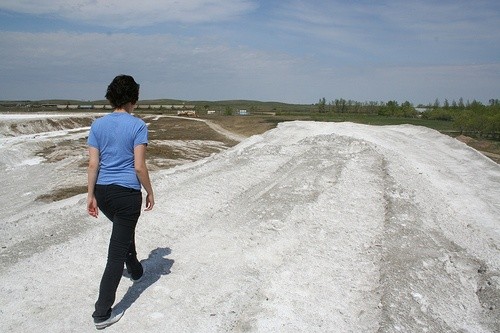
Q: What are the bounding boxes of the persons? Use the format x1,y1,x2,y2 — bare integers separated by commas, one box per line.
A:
86,75,154,330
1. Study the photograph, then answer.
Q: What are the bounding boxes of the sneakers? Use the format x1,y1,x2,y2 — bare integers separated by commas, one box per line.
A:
132,262,146,283
95,308,124,329
